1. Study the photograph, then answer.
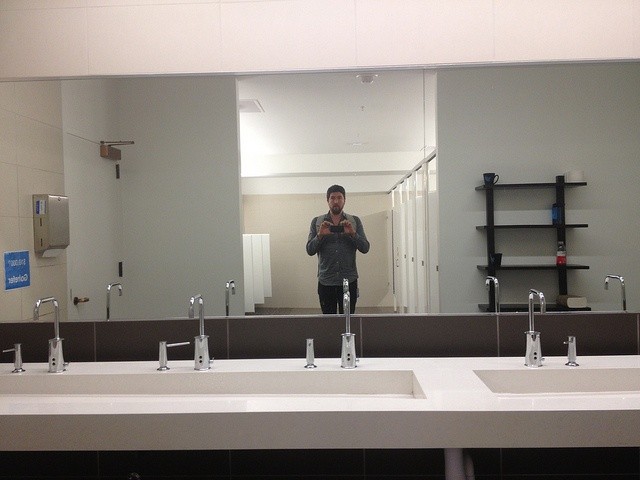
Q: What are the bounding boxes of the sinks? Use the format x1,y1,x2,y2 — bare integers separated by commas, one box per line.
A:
473,367,640,394
0,369,424,399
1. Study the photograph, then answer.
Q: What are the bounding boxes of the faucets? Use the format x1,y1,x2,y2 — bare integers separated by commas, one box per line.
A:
484,275,501,312
32,296,69,373
603,274,626,310
225,279,236,316
340,292,357,369
106,282,123,322
524,288,547,368
343,278,349,292
187,293,214,369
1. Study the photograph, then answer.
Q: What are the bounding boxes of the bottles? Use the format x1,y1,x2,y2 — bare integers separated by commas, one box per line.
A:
556,241,566,266
551,202,565,227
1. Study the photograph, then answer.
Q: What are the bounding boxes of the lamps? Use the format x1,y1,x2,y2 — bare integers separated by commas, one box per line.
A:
355,71,379,85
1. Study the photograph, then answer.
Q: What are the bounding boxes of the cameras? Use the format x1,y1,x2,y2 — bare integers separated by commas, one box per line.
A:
331,225,345,232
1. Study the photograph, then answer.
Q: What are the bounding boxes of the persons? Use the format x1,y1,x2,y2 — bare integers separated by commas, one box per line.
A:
306,184,370,315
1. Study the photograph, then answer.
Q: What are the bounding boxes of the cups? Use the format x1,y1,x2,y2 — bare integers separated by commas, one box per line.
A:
482,173,500,185
490,252,503,267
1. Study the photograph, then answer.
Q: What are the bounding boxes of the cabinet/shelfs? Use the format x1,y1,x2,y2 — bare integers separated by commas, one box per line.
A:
475,176,593,312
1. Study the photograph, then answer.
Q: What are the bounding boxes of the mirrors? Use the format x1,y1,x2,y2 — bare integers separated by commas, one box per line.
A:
237,66,440,316
